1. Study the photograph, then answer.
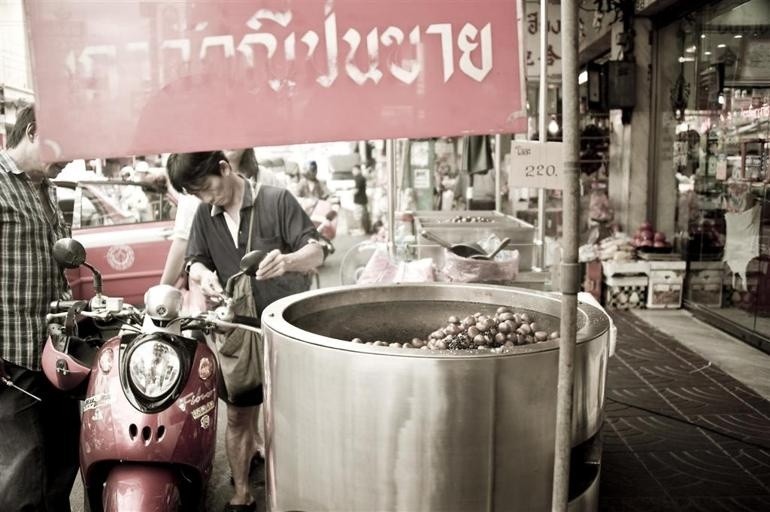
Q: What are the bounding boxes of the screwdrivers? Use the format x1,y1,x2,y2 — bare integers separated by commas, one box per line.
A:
1,377,42,402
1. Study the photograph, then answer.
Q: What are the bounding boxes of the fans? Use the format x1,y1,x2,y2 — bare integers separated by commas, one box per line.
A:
340,240,382,287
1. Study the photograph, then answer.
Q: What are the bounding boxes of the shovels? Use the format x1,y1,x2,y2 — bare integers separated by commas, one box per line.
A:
421,230,510,261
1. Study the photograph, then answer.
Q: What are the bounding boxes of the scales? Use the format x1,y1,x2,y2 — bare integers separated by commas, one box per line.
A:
588,218,611,245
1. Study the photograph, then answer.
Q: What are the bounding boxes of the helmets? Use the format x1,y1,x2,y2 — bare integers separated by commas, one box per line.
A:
42,318,106,391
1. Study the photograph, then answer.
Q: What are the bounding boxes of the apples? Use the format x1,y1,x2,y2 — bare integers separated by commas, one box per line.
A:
629,223,669,248
694,219,723,246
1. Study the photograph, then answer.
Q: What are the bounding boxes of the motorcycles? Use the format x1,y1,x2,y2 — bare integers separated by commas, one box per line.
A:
297,193,339,266
42,239,269,511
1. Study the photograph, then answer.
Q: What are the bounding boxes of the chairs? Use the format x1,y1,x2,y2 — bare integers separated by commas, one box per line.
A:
58,199,82,225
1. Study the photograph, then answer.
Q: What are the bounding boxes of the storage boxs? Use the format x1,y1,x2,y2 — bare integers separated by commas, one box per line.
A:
412,205,537,272
584,247,688,312
685,265,728,309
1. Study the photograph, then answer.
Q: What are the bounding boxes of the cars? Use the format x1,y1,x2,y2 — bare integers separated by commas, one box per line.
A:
50,179,179,306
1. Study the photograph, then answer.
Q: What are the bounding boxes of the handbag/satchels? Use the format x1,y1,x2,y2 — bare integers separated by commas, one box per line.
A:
211,268,264,406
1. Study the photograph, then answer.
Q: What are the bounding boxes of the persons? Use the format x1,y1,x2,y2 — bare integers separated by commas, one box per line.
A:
0,105,83,512
160,148,327,512
345,164,370,237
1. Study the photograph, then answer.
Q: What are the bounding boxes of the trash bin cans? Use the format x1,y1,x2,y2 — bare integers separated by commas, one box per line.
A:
340,189,363,235
